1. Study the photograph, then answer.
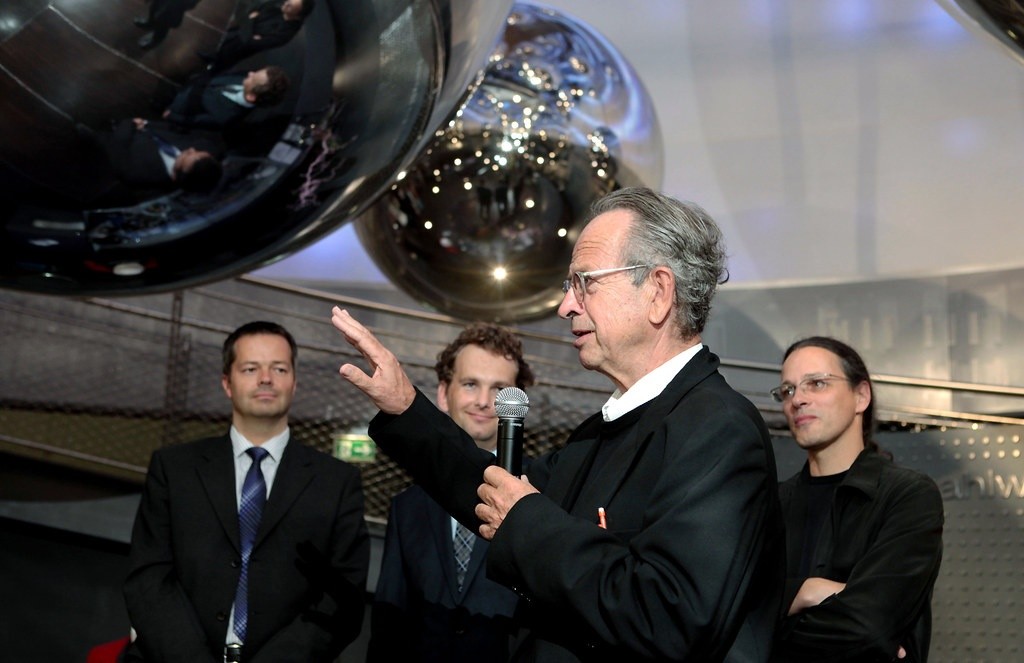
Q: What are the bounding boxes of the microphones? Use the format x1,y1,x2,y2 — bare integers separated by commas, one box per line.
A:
494,387,529,479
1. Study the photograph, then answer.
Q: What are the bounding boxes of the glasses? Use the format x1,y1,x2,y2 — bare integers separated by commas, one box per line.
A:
770,372,859,403
562,264,659,297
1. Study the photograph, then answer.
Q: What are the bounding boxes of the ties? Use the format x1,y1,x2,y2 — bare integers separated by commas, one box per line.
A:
232,446,269,646
452,520,476,592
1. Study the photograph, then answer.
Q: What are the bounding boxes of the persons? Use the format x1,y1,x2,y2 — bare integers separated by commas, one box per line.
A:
106,0,320,197
123,320,370,663
330,185,777,663
778,337,944,663
368,322,536,663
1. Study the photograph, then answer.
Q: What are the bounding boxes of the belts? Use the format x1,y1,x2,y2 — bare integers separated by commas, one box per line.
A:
222,643,241,663
